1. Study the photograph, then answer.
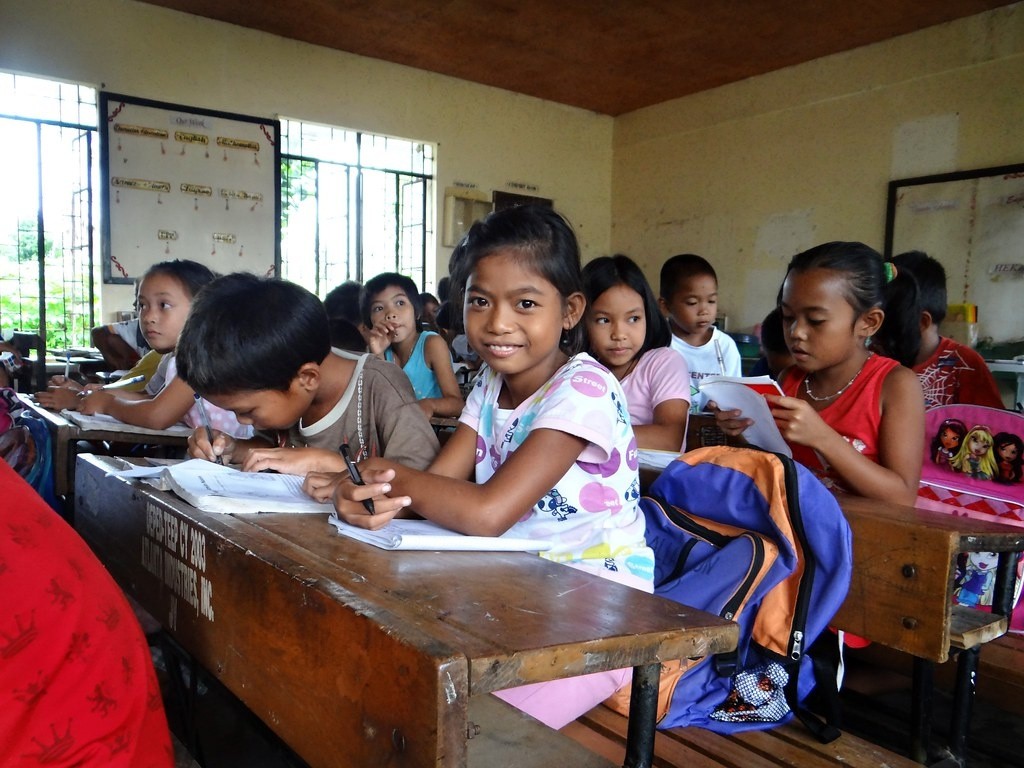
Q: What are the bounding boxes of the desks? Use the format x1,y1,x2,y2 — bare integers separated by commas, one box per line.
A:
1,347,1023,767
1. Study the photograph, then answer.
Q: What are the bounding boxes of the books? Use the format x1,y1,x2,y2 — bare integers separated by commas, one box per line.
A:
130,459,341,515
326,514,555,553
59,408,197,439
635,447,686,470
697,375,796,460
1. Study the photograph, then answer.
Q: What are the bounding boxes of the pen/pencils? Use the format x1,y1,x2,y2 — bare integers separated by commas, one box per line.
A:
65,353,70,381
714,339,728,377
76,374,145,397
193,393,223,464
339,444,376,516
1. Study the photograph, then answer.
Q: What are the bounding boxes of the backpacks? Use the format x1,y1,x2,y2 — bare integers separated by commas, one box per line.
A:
643,446,855,743
920,403,1023,636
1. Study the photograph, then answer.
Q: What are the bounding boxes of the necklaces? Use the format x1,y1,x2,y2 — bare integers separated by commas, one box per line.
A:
804,352,873,401
621,359,635,380
393,344,414,366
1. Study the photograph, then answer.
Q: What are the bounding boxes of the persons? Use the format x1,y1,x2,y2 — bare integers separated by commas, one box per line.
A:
706,241,925,709
566,245,1002,458
0,457,175,768
304,207,653,732
0,260,487,444
177,273,442,482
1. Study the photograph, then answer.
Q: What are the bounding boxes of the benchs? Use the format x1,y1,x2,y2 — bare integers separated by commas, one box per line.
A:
557,703,925,767
973,631,1023,688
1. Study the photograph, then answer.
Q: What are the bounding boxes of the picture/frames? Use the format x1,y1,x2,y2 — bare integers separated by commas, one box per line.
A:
883,162,1024,359
99,90,281,285
